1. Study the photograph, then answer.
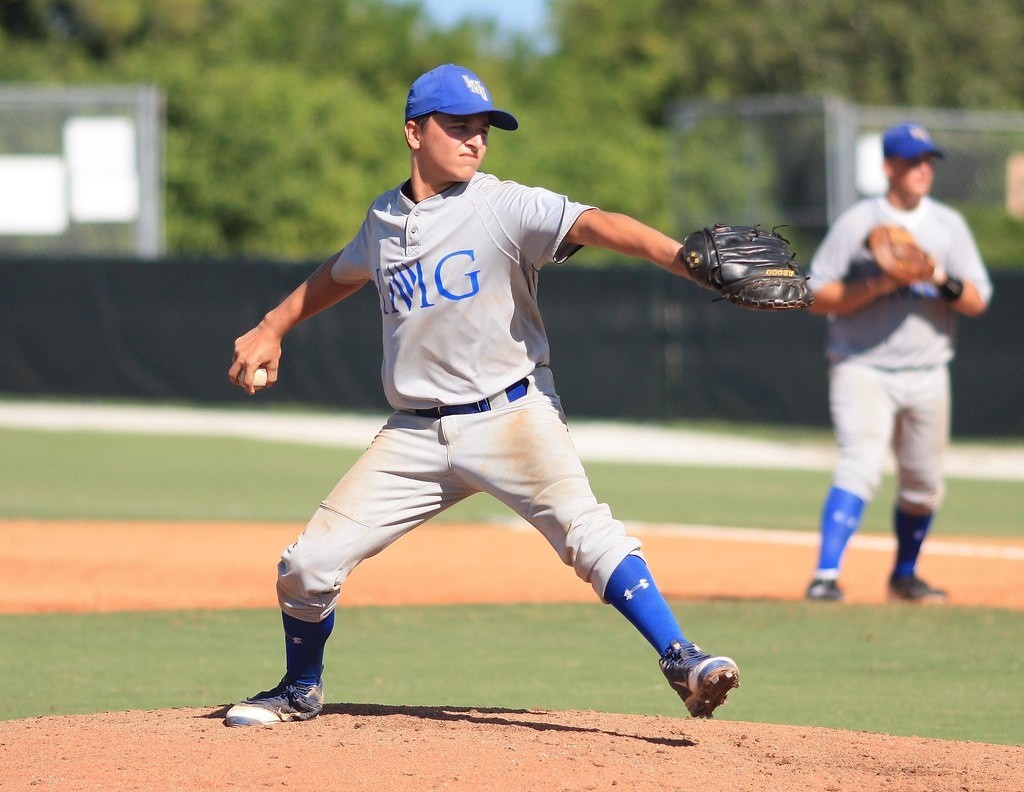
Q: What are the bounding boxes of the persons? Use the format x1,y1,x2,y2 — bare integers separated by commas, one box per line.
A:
223,62,813,728
804,123,995,606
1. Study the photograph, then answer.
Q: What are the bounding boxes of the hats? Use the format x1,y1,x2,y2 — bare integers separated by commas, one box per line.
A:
405,63,518,131
884,124,944,161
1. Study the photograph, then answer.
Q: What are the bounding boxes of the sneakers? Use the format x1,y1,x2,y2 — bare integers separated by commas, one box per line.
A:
658,640,741,719
226,673,324,725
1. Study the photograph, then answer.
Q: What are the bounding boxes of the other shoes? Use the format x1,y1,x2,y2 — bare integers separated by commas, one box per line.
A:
889,575,946,603
806,579,840,599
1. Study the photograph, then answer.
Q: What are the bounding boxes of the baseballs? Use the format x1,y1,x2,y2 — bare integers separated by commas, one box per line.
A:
238,365,268,392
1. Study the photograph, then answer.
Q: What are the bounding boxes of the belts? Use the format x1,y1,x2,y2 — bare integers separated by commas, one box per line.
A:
415,378,529,419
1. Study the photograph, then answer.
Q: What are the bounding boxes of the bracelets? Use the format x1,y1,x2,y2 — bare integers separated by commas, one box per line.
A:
937,271,963,303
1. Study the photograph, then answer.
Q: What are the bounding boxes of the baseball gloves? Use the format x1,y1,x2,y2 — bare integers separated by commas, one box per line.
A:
864,223,945,288
678,222,817,313
